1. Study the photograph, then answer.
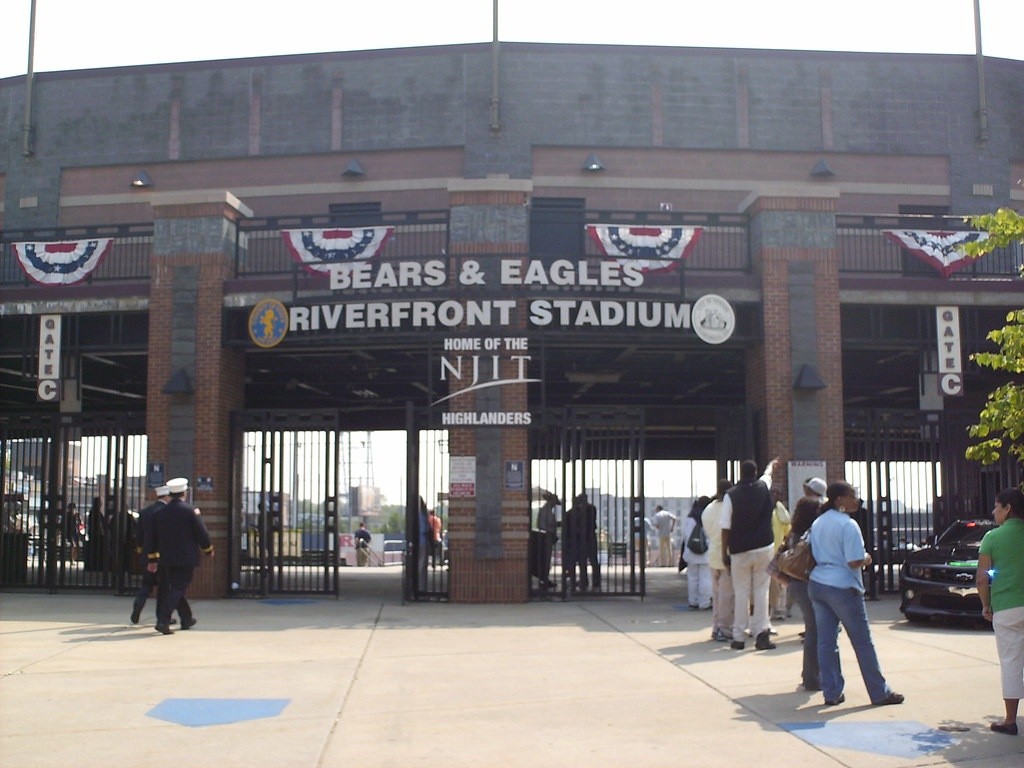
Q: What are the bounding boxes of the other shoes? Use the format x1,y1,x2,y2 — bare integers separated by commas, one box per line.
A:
731,640,745,651
873,692,904,705
769,613,787,622
155,623,173,634
130,603,143,624
711,628,726,641
802,676,822,692
755,632,776,650
169,618,176,625
991,722,1017,736
825,694,845,705
181,618,196,630
798,631,805,639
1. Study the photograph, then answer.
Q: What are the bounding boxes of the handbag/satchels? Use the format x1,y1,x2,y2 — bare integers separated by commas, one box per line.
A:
766,529,795,587
777,528,817,583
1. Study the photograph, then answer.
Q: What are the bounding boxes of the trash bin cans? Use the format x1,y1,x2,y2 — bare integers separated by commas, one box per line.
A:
0,533,32,586
531,528,556,582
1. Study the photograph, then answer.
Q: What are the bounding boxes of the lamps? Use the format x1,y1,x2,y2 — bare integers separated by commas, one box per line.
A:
585,154,605,172
163,365,194,402
341,159,364,181
128,168,152,187
796,364,829,391
812,159,834,179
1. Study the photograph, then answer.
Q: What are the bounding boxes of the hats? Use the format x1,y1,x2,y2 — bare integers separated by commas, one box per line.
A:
166,477,189,493
803,476,827,497
154,486,169,496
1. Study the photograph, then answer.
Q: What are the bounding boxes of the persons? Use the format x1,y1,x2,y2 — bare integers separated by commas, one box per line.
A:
131,486,176,625
808,481,905,706
539,492,561,587
976,488,1024,735
700,479,734,641
803,477,827,501
633,511,654,567
152,477,214,635
721,458,792,649
354,522,371,567
654,506,677,567
66,502,82,565
785,496,823,691
681,496,713,610
420,496,441,591
85,495,136,584
567,493,599,587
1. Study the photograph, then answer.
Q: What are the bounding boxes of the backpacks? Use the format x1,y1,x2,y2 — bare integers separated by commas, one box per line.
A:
687,517,709,554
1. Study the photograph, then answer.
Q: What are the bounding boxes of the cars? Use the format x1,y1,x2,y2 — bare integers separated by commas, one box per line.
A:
897,519,1001,623
